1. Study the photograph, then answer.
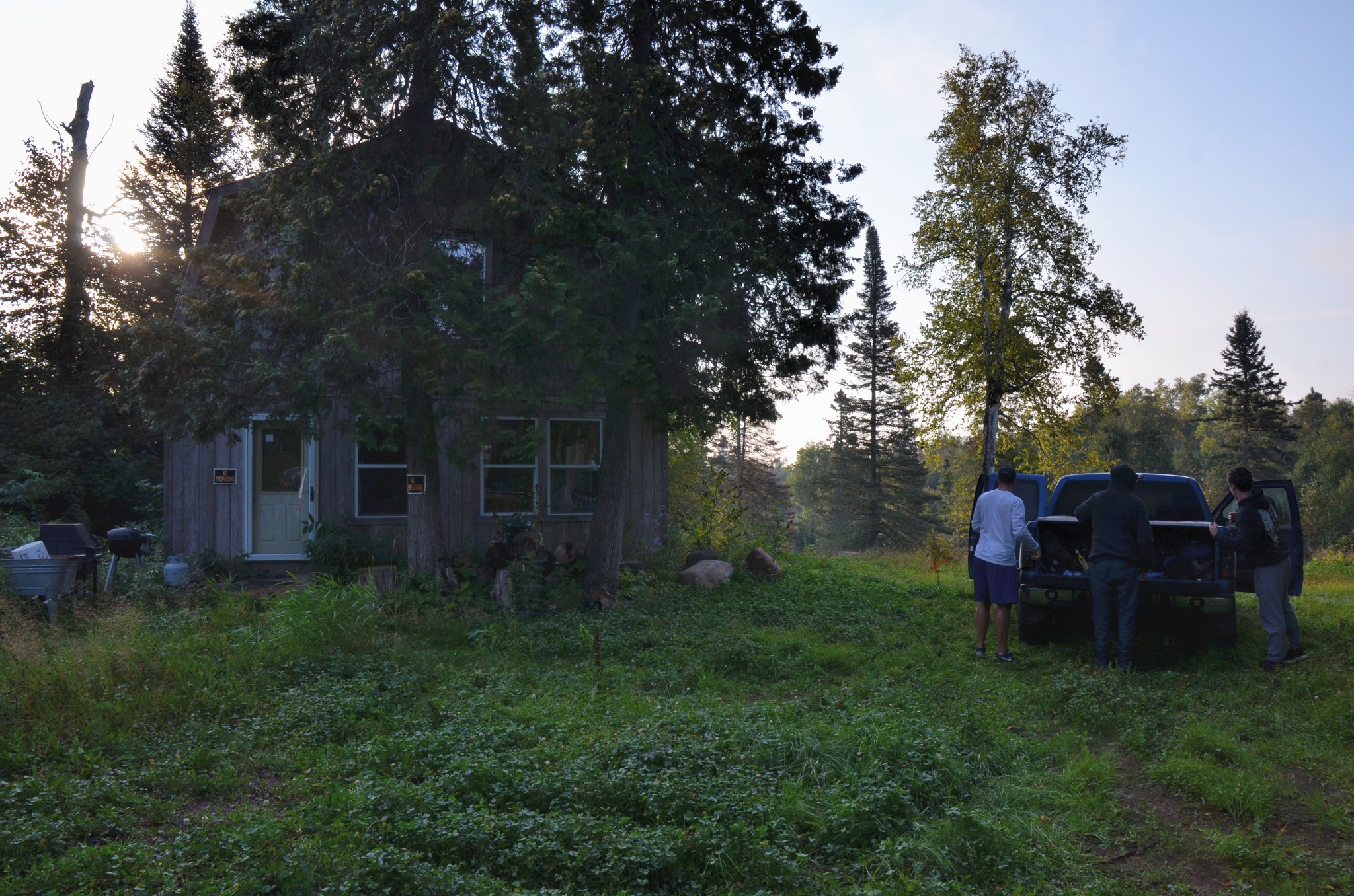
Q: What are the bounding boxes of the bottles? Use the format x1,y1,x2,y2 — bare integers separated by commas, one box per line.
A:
1076,550,1088,570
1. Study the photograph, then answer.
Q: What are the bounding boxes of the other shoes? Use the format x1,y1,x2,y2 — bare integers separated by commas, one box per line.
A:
1259,660,1285,672
1283,650,1308,665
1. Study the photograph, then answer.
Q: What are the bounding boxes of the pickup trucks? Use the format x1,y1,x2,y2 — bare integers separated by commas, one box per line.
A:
967,472,1304,651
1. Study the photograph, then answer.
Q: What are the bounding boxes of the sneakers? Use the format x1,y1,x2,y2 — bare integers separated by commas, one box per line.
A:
975,647,985,657
994,650,1014,662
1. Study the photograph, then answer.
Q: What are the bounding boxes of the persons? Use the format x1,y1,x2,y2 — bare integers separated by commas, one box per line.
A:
970,466,1041,663
1078,464,1153,672
1209,467,1309,670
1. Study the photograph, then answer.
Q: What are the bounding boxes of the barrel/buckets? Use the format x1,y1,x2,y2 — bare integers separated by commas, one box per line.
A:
11,541,50,559
503,512,532,547
